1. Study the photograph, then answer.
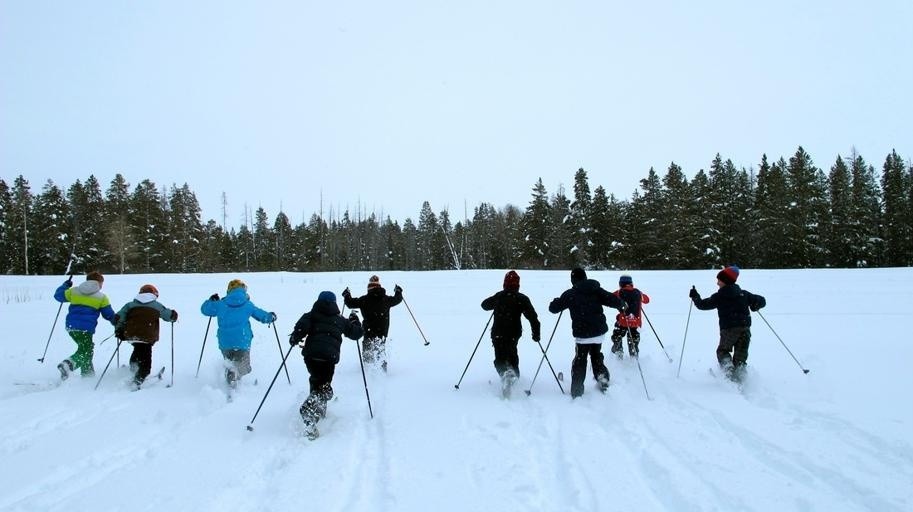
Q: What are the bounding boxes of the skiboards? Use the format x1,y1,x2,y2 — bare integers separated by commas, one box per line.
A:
141,366,166,388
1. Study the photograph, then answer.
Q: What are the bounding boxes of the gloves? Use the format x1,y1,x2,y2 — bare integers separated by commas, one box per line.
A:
170,309,178,323
689,288,699,299
619,303,631,317
64,279,73,288
341,286,351,298
270,311,278,322
289,332,302,346
349,313,359,322
532,333,541,342
393,284,404,293
210,294,220,301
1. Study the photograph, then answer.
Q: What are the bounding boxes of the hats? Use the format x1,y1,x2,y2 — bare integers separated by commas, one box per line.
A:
317,291,338,305
716,264,740,286
619,273,633,284
227,279,248,292
502,271,520,289
570,267,587,284
139,284,159,298
366,274,381,291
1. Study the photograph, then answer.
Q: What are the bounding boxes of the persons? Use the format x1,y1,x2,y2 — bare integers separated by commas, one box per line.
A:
479,269,543,398
288,289,365,443
607,274,651,360
548,267,629,402
686,265,766,392
199,277,276,399
339,274,403,376
51,272,118,383
113,282,178,388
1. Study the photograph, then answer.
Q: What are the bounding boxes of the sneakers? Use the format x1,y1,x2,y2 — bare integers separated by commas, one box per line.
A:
56,361,71,382
